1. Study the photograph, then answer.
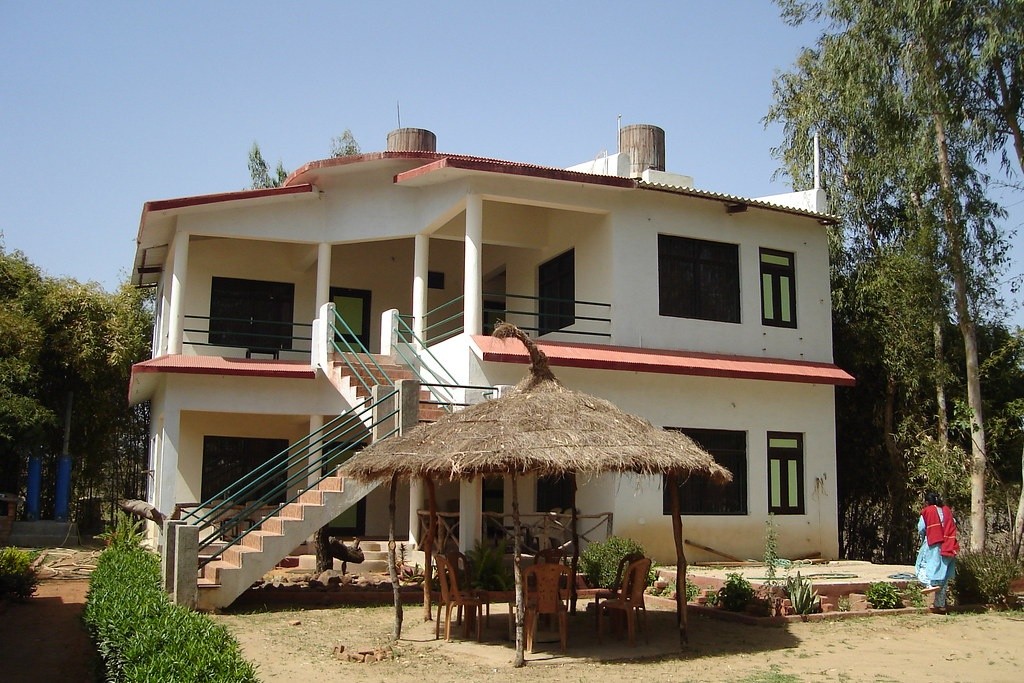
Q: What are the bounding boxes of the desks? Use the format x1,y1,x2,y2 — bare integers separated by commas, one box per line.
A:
246,346,280,361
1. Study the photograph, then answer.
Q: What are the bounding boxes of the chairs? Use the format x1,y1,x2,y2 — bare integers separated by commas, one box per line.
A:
520,549,576,652
434,551,490,643
596,554,651,647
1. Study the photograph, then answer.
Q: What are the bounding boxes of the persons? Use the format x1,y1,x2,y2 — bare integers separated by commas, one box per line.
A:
915,493,956,612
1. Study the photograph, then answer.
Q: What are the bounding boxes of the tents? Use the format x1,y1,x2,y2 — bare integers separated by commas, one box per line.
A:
338,323,733,667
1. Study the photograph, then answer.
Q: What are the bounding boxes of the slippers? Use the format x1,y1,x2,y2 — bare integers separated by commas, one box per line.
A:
930,604,946,613
921,585,941,593
